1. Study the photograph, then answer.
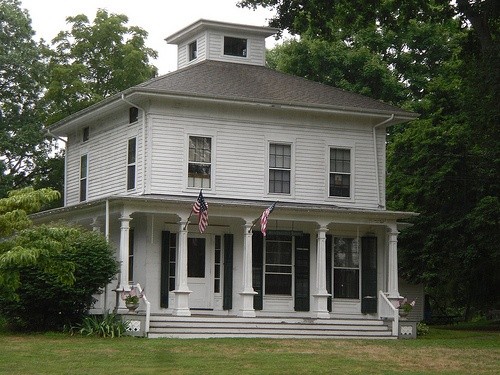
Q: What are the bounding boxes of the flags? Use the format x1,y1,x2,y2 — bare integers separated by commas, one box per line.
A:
260,202,275,237
191,191,208,234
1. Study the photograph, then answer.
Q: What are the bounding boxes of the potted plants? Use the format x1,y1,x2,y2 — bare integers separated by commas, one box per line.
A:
397,304,418,339
121,295,143,336
